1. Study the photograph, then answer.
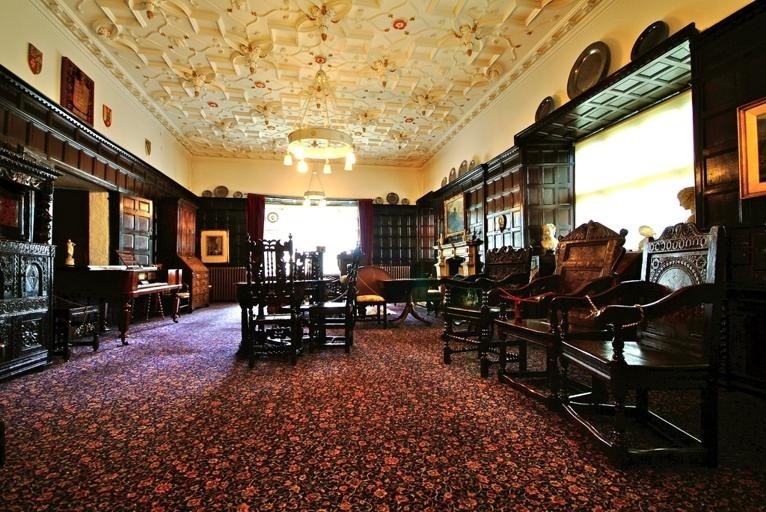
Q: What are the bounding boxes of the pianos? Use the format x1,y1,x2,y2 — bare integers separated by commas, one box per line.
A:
54,265,183,347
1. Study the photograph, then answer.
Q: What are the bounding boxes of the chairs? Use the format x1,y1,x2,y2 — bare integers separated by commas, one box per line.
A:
442,245,529,376
496,219,628,405
354,266,393,329
233,232,359,363
550,221,728,467
153,282,187,316
51,286,99,359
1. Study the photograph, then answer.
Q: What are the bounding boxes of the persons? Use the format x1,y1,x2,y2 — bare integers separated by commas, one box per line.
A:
541,224,559,249
678,187,696,222
67,240,76,259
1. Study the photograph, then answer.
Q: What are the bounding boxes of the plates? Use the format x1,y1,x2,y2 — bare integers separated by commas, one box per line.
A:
567,41,608,101
441,160,475,187
534,96,554,120
630,21,669,60
375,193,411,205
202,186,243,198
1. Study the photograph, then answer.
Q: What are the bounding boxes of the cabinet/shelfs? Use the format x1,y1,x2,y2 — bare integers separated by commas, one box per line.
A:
159,196,210,315
359,141,575,292
2,146,53,384
121,194,154,268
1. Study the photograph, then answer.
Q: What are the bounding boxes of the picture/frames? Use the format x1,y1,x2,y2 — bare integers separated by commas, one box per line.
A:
735,95,766,202
198,229,229,265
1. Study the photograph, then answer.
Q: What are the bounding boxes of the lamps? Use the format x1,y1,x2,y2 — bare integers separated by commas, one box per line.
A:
282,54,357,175
304,168,327,211
